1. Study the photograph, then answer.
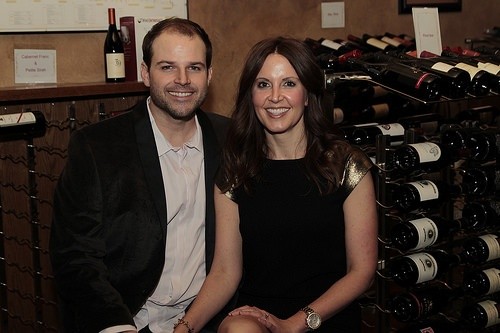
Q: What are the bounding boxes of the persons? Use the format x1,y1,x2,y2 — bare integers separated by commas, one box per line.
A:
171,36,381,333
48,16,244,333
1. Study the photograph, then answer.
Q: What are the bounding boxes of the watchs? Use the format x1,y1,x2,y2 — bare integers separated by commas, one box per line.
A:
301,305,322,330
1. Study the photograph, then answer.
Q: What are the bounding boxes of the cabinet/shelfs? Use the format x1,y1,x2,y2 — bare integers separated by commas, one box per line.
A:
0,76,150,333
302,32,500,333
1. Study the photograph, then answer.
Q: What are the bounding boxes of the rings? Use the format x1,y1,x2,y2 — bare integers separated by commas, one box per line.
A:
264,314,269,320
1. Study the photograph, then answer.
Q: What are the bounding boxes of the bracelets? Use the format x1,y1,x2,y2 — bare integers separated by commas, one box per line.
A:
173,317,195,333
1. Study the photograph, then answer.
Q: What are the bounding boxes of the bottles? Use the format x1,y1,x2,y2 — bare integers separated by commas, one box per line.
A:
103,7,127,82
110,103,140,117
0,111,47,142
302,26,499,333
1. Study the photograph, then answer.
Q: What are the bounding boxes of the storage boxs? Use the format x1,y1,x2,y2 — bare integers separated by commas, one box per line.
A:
119,14,165,82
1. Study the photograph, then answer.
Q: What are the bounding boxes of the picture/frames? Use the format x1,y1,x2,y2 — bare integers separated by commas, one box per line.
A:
397,0,463,16
1,0,188,35
